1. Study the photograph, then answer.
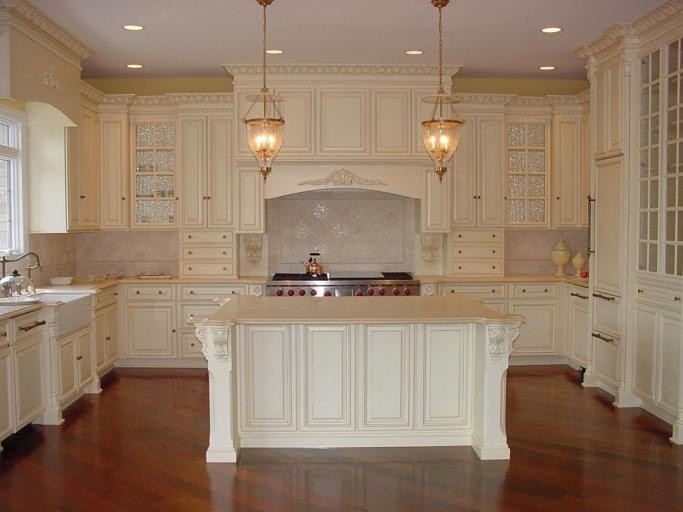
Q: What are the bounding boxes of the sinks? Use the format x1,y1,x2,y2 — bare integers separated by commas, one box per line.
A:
18,293,90,338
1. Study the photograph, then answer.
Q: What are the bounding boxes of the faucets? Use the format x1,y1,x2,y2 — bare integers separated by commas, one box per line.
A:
0,251,44,292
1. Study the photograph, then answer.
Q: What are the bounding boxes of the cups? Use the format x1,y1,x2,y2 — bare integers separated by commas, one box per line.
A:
155,189,174,197
168,215,174,223
136,165,156,172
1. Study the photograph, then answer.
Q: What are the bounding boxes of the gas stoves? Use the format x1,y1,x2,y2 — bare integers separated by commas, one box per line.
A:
266,271,420,297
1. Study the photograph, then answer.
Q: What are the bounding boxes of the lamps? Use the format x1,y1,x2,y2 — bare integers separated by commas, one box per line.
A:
236,2,288,183
416,1,466,183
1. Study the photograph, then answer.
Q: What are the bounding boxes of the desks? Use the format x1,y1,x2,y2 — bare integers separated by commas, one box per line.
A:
192,293,526,467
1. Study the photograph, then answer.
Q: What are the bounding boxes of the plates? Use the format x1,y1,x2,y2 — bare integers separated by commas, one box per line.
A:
0,296,36,306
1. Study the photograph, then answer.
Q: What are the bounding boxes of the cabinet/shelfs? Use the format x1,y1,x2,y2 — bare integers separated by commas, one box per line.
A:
630,2,682,294
438,284,508,313
98,92,134,235
176,281,247,369
0,1,88,129
28,81,100,235
174,93,236,230
0,314,14,444
130,95,178,234
412,63,465,161
175,232,242,281
444,229,504,279
449,92,517,228
9,306,47,434
580,24,629,161
626,284,682,446
233,161,264,233
589,155,628,299
556,283,589,375
314,65,413,161
506,281,561,365
93,282,121,374
551,91,589,229
420,165,447,233
223,62,314,162
55,323,94,406
502,96,551,229
581,292,628,410
122,280,176,367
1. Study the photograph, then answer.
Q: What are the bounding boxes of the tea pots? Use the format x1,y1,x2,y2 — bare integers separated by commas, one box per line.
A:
299,253,322,277
